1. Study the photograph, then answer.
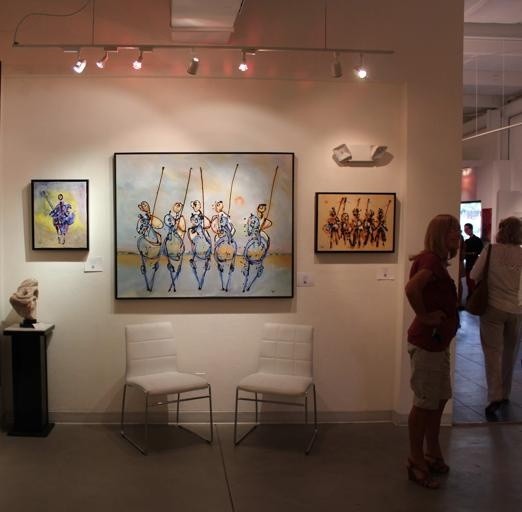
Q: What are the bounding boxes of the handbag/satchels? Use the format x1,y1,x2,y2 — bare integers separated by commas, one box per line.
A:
464,272,489,316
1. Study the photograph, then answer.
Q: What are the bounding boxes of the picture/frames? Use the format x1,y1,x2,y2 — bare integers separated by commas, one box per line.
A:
113,151,295,300
30,178,90,252
313,191,397,255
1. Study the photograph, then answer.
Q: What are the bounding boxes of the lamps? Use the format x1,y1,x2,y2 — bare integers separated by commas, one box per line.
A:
95,50,110,70
331,51,343,80
131,50,144,70
70,48,88,75
353,53,368,80
237,51,250,74
187,46,201,75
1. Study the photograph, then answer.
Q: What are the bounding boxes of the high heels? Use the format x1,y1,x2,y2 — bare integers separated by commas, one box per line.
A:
407,458,440,489
426,455,448,475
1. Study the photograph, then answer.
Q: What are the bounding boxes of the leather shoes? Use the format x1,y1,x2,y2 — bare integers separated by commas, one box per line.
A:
501,398,509,404
485,400,501,416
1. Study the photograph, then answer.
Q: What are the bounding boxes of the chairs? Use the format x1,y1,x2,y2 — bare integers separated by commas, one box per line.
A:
233,321,317,454
119,320,213,455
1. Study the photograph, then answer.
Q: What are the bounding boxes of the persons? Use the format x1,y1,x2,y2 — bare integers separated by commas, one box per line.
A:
455,235,468,310
465,224,483,305
469,217,521,419
405,215,461,489
